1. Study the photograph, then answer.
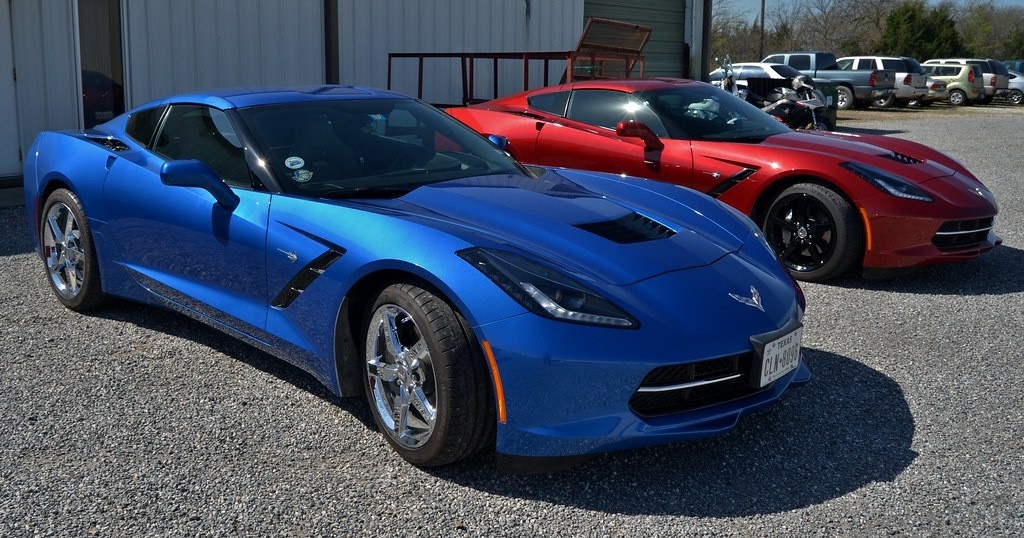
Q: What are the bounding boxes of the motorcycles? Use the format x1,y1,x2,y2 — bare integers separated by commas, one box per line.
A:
679,57,835,132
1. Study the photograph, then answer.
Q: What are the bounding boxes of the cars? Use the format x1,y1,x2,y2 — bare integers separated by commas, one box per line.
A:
23,84,813,469
924,59,1012,104
424,78,1003,282
709,62,805,92
993,70,1024,106
822,56,929,108
1001,60,1024,73
919,64,985,106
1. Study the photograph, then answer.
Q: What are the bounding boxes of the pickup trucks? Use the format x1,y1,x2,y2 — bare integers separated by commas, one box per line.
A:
760,51,899,111
